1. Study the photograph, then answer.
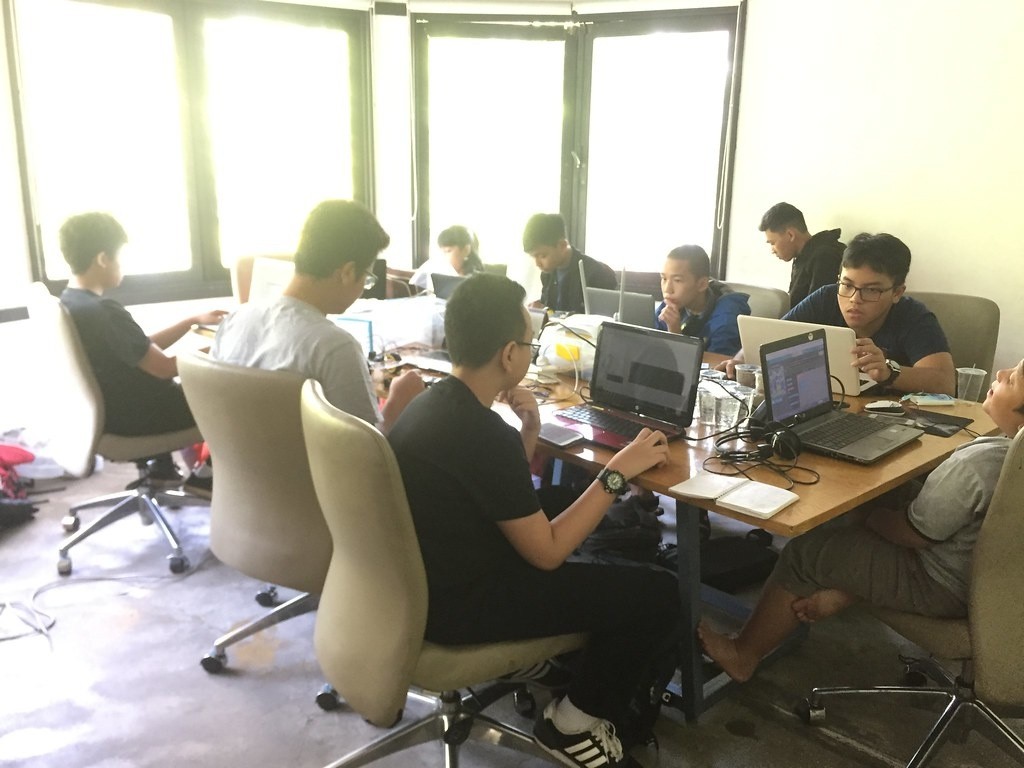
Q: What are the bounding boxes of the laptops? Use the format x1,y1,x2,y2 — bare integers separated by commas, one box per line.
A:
539,321,704,452
431,273,466,301
198,257,295,332
737,314,877,397
587,287,655,329
759,328,926,464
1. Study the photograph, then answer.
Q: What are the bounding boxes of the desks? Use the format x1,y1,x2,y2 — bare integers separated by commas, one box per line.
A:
122,297,1006,726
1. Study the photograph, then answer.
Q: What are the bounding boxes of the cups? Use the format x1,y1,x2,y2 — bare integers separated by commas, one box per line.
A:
956,367,987,407
691,364,763,432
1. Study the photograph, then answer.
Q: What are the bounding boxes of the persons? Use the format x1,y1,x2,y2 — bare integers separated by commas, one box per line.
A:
653,245,752,355
386,271,686,768
59,211,230,502
758,202,847,308
695,354,1024,681
712,232,956,397
416,224,485,297
206,198,428,436
522,213,618,319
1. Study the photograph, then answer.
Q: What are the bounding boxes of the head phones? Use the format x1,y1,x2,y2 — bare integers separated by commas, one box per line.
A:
714,420,801,460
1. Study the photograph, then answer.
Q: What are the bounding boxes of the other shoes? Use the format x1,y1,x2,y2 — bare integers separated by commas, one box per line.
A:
183,473,214,498
146,464,185,487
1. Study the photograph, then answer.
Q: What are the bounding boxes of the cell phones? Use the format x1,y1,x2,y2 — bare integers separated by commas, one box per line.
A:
539,423,585,450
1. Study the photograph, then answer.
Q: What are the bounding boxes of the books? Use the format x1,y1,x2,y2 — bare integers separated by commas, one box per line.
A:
668,470,800,520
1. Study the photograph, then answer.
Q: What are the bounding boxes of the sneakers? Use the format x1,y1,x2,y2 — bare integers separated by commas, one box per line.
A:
495,658,574,689
532,698,643,768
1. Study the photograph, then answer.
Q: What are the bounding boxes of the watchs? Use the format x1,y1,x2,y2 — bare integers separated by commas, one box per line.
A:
544,307,549,310
595,469,630,496
880,359,900,386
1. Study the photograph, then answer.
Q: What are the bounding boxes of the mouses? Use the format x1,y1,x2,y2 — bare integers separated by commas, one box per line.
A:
864,400,905,416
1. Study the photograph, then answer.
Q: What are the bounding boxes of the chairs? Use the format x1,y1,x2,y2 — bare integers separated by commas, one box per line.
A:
24,281,593,768
479,264,1000,406
798,425,1024,768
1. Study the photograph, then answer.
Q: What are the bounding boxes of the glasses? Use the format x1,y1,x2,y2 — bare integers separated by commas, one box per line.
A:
836,277,894,301
351,261,378,289
507,342,541,356
